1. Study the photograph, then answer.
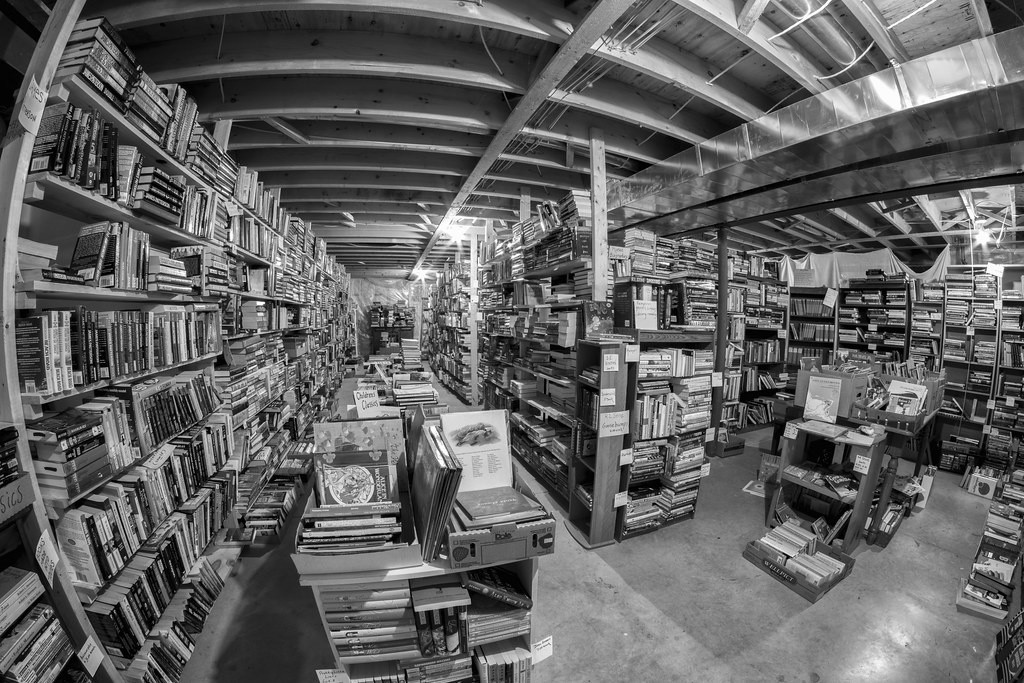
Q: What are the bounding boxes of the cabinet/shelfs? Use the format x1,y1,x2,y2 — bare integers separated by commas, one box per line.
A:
0,0,1024,683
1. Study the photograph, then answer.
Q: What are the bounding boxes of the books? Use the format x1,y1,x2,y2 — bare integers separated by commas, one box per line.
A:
804,375,842,424
0,17,1024,683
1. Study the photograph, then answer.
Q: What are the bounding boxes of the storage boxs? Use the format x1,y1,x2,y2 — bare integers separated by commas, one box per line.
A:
743,537,855,604
795,368,868,418
276,455,314,483
445,462,557,571
290,489,424,576
851,399,927,434
716,435,745,458
287,441,314,461
254,496,301,544
880,372,946,415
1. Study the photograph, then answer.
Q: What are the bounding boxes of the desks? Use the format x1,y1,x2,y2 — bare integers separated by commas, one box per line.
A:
848,401,945,545
372,325,414,353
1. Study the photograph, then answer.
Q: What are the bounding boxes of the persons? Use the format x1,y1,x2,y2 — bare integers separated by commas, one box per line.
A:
974,583,1000,603
